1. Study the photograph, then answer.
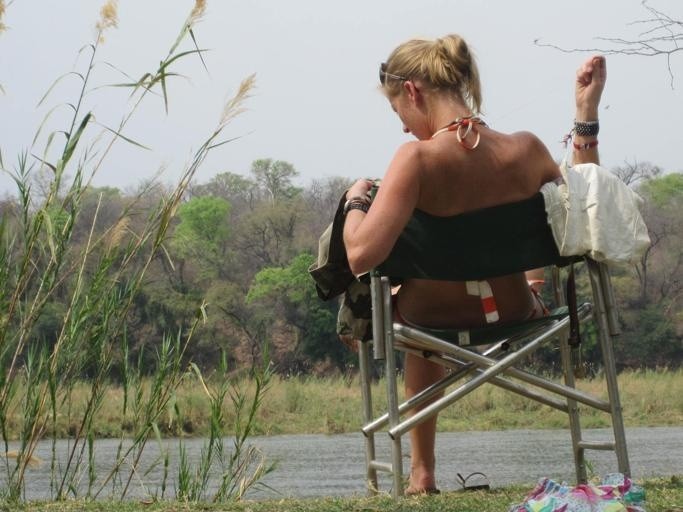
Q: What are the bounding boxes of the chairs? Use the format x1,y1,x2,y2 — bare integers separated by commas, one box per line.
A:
358,196,633,495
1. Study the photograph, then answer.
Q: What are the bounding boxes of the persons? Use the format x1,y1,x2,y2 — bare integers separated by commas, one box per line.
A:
338,32,606,498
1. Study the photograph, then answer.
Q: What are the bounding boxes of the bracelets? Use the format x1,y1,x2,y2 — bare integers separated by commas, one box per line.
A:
342,195,369,214
572,141,598,151
569,119,600,139
